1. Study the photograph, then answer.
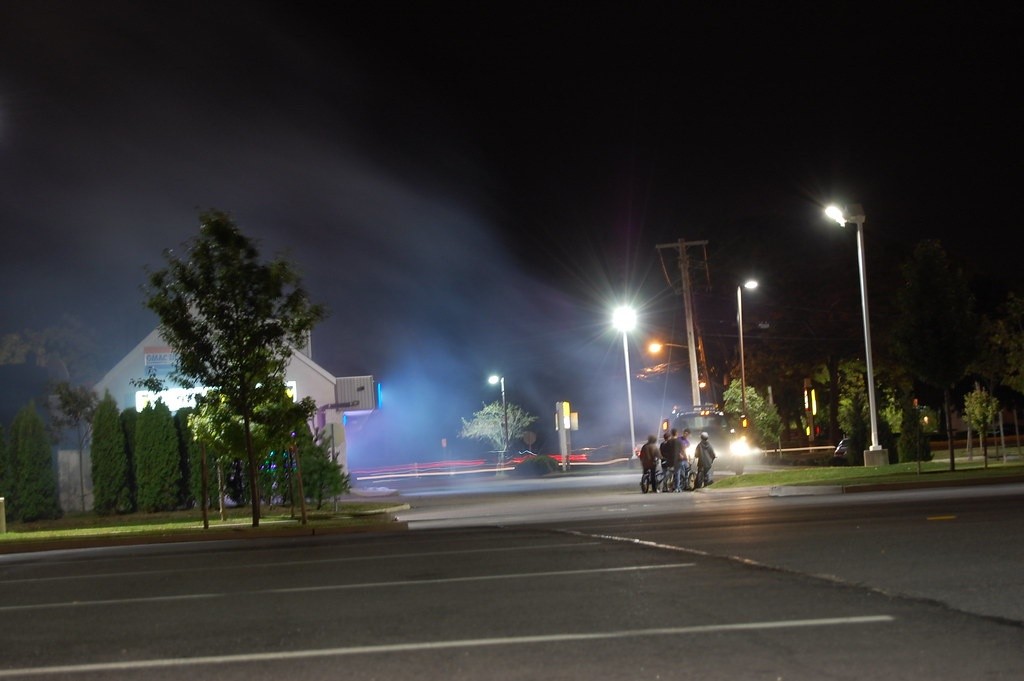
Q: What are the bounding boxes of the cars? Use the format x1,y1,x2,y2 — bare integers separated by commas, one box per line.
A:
834,438,850,455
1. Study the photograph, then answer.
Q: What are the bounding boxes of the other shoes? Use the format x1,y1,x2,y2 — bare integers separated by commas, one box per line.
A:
653,486,696,493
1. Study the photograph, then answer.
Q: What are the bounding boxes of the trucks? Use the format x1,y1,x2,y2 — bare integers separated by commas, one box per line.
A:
658,408,744,476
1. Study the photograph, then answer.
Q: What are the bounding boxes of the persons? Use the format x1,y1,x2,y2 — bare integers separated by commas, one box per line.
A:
640,434,665,493
677,429,690,448
695,432,717,484
660,434,674,481
664,429,689,493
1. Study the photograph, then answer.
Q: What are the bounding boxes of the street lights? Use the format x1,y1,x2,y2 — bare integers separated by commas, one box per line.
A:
486,376,510,451
649,336,710,390
737,280,758,416
824,204,883,451
610,305,638,461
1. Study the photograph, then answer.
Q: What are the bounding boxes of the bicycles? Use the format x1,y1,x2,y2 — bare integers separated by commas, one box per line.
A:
664,455,697,493
642,458,666,494
